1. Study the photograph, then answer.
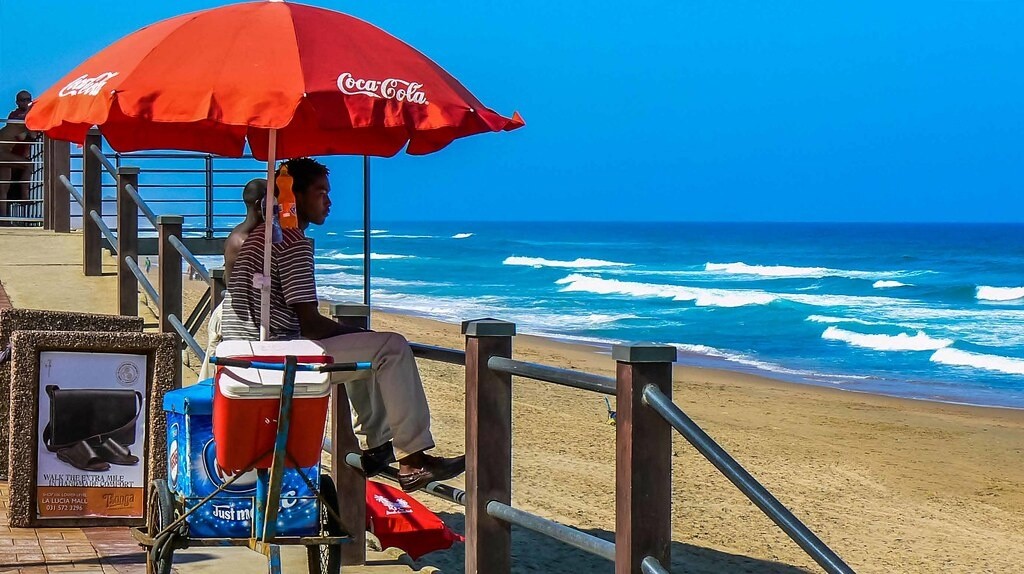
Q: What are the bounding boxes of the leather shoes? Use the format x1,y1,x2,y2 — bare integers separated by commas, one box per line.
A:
398,454,464,491
359,441,398,477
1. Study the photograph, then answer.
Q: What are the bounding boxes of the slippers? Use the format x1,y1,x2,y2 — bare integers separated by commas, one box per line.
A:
91,438,140,466
57,441,111,471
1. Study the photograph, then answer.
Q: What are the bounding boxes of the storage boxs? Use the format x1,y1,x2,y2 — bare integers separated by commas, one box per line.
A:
210,338,334,472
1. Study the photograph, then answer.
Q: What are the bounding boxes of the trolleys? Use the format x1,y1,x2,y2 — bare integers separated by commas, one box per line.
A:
131,356,372,573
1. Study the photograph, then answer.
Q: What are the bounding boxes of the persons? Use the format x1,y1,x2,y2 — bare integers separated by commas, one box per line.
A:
221,158,465,492
8,91,42,216
0,116,40,220
199,180,265,383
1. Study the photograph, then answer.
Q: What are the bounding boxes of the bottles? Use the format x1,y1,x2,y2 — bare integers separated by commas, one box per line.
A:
260,194,283,244
277,165,299,229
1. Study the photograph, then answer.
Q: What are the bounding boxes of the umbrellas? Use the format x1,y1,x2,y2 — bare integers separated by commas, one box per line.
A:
25,1,524,343
